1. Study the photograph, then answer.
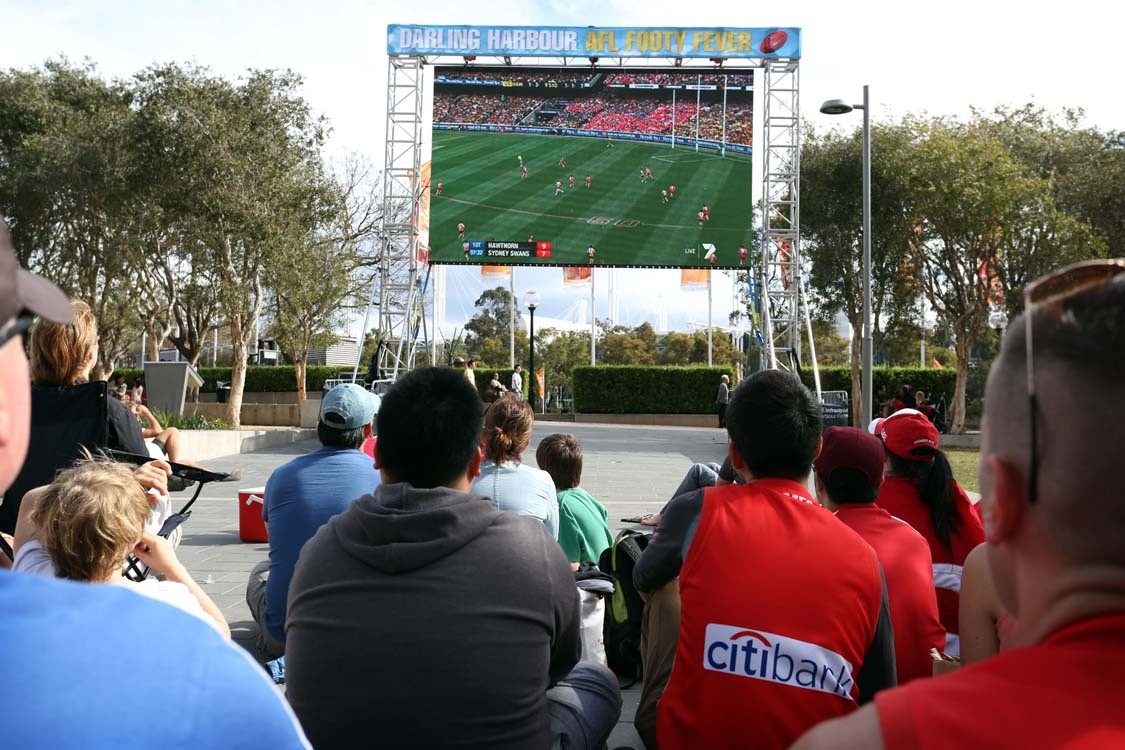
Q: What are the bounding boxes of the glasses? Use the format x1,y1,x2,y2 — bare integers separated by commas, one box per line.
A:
0,306,34,347
1025,257,1124,504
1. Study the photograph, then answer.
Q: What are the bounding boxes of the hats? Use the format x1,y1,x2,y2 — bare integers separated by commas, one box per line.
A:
867,407,940,464
0,215,73,327
811,426,887,492
319,383,382,430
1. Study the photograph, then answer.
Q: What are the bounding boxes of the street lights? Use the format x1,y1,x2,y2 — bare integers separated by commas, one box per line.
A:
523,289,540,413
820,99,873,432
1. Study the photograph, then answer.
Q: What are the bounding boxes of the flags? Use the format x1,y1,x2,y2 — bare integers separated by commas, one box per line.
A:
681,267,712,292
563,266,592,285
481,264,512,280
407,157,441,276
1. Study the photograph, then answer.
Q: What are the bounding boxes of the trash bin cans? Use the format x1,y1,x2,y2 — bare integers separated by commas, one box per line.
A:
216,386,231,402
821,404,848,433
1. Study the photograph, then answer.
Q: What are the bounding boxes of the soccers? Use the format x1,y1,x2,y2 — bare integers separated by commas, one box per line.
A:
570,208,574,214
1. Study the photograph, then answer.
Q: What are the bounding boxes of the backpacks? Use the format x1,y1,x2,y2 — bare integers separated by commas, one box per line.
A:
596,528,655,681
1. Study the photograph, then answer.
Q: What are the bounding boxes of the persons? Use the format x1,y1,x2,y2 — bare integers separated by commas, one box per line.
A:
788,260,1125,750
862,408,996,659
956,540,1023,669
245,382,382,658
535,434,614,581
430,71,753,268
805,425,945,694
878,384,942,423
10,298,150,575
283,365,623,750
12,443,232,661
0,213,305,749
103,374,179,463
461,349,560,553
633,370,897,750
641,374,749,540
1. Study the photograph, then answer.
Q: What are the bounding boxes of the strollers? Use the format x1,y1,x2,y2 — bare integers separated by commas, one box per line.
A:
921,391,949,434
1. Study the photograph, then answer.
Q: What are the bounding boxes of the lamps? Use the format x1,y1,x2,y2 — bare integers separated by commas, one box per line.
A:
710,57,727,67
463,56,476,66
589,57,598,65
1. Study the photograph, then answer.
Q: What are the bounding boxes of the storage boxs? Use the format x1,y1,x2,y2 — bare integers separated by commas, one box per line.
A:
238,487,269,543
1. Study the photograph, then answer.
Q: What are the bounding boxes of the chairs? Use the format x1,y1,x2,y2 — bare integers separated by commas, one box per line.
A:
925,393,950,433
0,378,236,581
